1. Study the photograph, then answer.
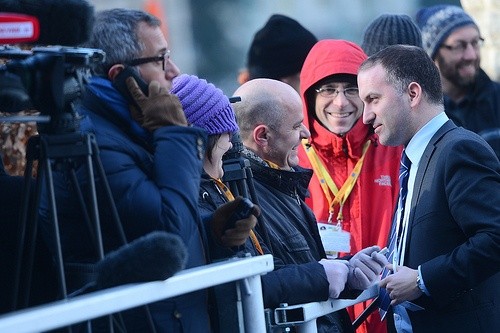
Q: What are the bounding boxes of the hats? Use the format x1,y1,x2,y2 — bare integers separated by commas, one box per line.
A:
416,5,478,59
360,15,423,54
167,74,239,133
247,13,319,79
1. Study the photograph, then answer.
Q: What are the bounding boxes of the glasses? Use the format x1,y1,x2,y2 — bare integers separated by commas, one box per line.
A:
441,34,485,53
313,86,361,99
125,50,171,71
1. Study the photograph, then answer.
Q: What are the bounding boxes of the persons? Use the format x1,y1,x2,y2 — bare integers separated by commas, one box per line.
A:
416,4,500,160
361,14,423,60
230,78,388,333
32,9,261,333
0,0,189,333
356,45,500,333
170,73,349,308
295,39,405,333
238,14,320,94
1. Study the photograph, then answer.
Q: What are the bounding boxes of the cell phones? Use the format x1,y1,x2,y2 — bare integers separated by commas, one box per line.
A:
110,65,150,114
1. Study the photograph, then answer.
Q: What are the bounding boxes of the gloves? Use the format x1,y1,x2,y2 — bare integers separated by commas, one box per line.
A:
319,258,350,300
347,246,388,293
128,76,188,132
210,194,261,246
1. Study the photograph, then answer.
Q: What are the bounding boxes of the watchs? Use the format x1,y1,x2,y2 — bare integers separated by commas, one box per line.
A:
415,274,425,293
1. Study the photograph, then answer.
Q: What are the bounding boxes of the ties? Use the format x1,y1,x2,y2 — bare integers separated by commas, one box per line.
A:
375,149,412,322
